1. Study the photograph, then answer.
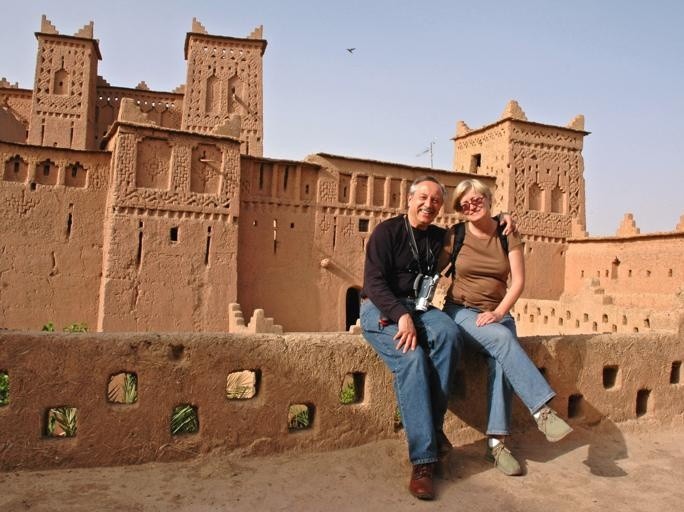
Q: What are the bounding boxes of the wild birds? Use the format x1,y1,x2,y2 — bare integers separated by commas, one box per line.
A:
345,48,355,54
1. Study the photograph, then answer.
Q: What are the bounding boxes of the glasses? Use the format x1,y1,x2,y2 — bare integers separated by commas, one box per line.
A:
462,196,485,210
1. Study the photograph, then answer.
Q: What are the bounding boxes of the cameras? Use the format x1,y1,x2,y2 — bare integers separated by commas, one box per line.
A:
413,273,440,313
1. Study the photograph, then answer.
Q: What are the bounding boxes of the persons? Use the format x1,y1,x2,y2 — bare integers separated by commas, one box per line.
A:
443,172,574,476
359,175,464,502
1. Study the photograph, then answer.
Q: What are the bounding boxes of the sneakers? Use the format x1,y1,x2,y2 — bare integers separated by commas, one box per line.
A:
536,405,573,441
486,439,521,475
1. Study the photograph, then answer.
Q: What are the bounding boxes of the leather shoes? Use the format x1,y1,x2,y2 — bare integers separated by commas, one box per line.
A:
409,429,452,499
